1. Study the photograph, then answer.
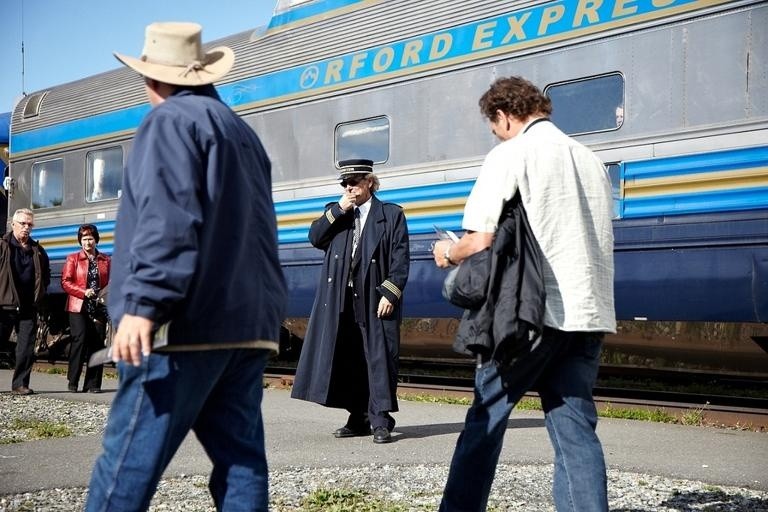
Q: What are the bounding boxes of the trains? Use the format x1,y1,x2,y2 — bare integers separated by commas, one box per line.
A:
0,0,768,378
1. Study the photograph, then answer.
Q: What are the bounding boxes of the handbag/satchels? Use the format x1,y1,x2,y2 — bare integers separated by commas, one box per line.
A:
88,299,108,325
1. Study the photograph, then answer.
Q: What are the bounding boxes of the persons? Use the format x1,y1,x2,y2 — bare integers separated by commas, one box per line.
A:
432,76,616,512
61,224,111,393
615,105,623,127
291,159,409,443
84,23,287,512
0,208,51,394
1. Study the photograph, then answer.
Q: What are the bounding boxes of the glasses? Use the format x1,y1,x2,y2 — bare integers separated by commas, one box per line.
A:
19,222,33,226
340,176,364,188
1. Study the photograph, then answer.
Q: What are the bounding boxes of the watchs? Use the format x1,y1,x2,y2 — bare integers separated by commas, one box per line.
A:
444,242,456,265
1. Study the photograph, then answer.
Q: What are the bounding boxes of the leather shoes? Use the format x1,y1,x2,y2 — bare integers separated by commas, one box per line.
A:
335,426,370,438
12,386,33,394
373,426,391,443
83,387,102,392
69,382,77,393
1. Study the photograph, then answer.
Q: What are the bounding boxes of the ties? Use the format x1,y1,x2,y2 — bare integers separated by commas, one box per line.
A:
354,208,360,245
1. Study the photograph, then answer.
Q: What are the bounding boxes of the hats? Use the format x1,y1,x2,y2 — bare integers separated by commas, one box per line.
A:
337,159,373,180
113,23,235,86
